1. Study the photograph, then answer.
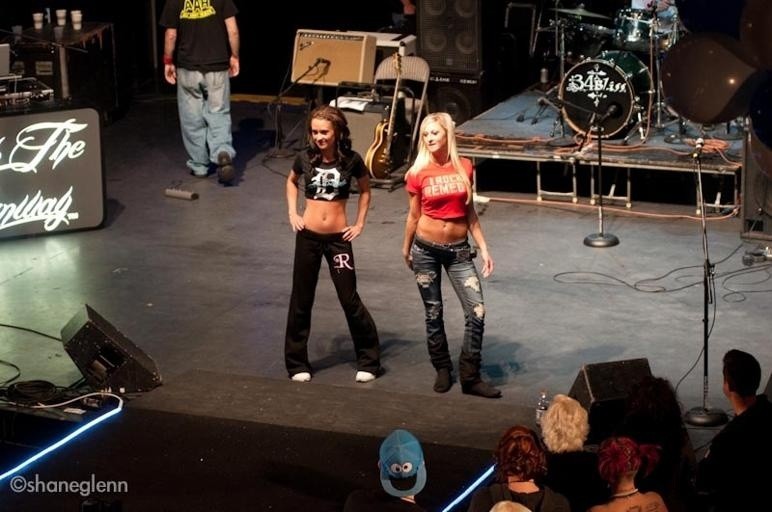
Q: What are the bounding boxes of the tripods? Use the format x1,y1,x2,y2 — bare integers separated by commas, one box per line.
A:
622,40,709,145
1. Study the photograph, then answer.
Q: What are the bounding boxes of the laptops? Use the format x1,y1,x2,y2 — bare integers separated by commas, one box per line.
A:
0,44,11,76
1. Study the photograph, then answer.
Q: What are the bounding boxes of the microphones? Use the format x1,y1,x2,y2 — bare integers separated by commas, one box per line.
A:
693,137,704,159
316,57,330,64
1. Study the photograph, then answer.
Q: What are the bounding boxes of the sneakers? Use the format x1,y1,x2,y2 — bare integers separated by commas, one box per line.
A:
355,370,375,382
217,152,236,185
433,368,452,393
462,381,502,397
291,371,311,383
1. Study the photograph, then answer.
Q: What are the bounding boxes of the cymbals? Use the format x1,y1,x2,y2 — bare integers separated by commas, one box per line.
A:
552,7,608,20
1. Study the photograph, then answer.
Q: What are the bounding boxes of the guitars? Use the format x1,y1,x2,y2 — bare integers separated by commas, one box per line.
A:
365,52,403,179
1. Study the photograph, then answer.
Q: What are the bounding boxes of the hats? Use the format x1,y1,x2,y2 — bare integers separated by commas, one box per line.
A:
379,427,428,497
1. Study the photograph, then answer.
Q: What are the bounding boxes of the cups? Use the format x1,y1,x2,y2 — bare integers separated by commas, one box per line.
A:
32,12,43,29
70,10,83,30
55,9,67,26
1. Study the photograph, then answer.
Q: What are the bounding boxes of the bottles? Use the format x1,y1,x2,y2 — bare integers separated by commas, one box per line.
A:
535,391,552,428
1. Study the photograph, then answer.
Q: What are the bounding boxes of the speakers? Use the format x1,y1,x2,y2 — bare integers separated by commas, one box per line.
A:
567,358,654,421
60,304,162,394
738,110,772,241
416,0,483,80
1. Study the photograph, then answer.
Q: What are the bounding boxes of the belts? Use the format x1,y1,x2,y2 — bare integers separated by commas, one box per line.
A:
414,239,456,259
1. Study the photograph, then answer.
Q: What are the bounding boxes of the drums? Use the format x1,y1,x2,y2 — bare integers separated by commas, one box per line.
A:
558,49,654,139
614,8,657,50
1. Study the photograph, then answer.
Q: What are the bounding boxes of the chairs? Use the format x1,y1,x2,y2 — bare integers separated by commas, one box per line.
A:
366,54,430,160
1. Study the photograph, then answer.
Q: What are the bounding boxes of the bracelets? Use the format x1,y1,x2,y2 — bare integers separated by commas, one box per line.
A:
163,55,173,63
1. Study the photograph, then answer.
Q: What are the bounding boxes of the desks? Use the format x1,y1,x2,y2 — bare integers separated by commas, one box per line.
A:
0,16,116,100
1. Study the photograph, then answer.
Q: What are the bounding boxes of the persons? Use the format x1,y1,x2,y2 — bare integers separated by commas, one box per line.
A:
284,106,381,382
342,349,772,512
158,0,240,184
402,113,501,398
